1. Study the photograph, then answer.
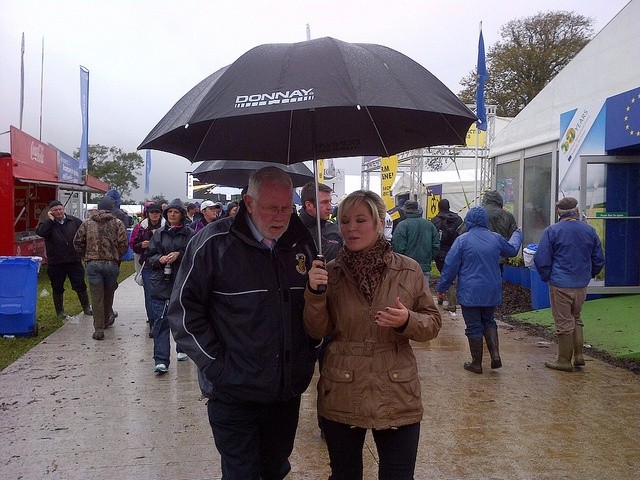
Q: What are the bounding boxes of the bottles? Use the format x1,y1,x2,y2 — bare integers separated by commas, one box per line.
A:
164,262,172,280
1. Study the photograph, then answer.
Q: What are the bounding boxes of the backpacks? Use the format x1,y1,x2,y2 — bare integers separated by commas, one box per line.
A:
439,217,462,248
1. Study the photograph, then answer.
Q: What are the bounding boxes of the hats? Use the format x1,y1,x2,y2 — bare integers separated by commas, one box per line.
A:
396,199,406,208
146,203,163,212
401,201,423,214
200,200,220,209
98,196,114,211
106,189,122,205
48,201,63,209
143,202,155,215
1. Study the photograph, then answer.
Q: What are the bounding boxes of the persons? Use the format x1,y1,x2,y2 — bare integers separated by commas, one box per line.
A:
129,201,157,324
193,202,201,221
435,205,525,375
73,196,129,340
143,197,198,374
195,200,221,233
100,189,135,318
382,212,394,243
131,203,168,338
224,202,238,218
302,189,442,480
213,201,225,220
533,197,606,373
457,189,519,279
166,166,326,480
390,199,441,289
296,181,345,441
184,203,197,226
430,199,465,313
35,200,93,325
390,200,409,235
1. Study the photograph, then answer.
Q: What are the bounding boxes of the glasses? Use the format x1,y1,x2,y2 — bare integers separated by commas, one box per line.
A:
252,199,294,215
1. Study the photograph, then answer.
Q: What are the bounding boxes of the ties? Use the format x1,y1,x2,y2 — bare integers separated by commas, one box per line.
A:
217,213,220,219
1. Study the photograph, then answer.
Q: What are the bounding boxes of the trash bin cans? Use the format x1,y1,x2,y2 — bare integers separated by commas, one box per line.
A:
523,244,551,309
0,255,42,339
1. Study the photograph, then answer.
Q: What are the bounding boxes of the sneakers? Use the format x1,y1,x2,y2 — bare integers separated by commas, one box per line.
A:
153,364,168,374
105,315,115,328
177,352,188,361
112,310,118,317
149,324,155,337
92,331,105,340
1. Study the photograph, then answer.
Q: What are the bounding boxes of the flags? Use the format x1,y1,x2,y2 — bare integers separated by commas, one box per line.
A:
145,148,151,196
316,159,327,185
78,65,90,170
475,24,490,132
380,154,401,221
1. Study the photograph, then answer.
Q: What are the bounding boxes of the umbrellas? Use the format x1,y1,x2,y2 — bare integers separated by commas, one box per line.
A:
189,159,316,190
135,23,483,293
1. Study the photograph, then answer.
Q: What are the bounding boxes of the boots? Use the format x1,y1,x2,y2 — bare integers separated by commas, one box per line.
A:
464,339,483,373
443,283,457,312
544,332,575,371
77,290,93,315
573,324,585,365
53,294,66,320
484,328,502,370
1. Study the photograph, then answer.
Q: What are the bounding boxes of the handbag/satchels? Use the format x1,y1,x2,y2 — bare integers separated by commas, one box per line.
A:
135,261,147,286
